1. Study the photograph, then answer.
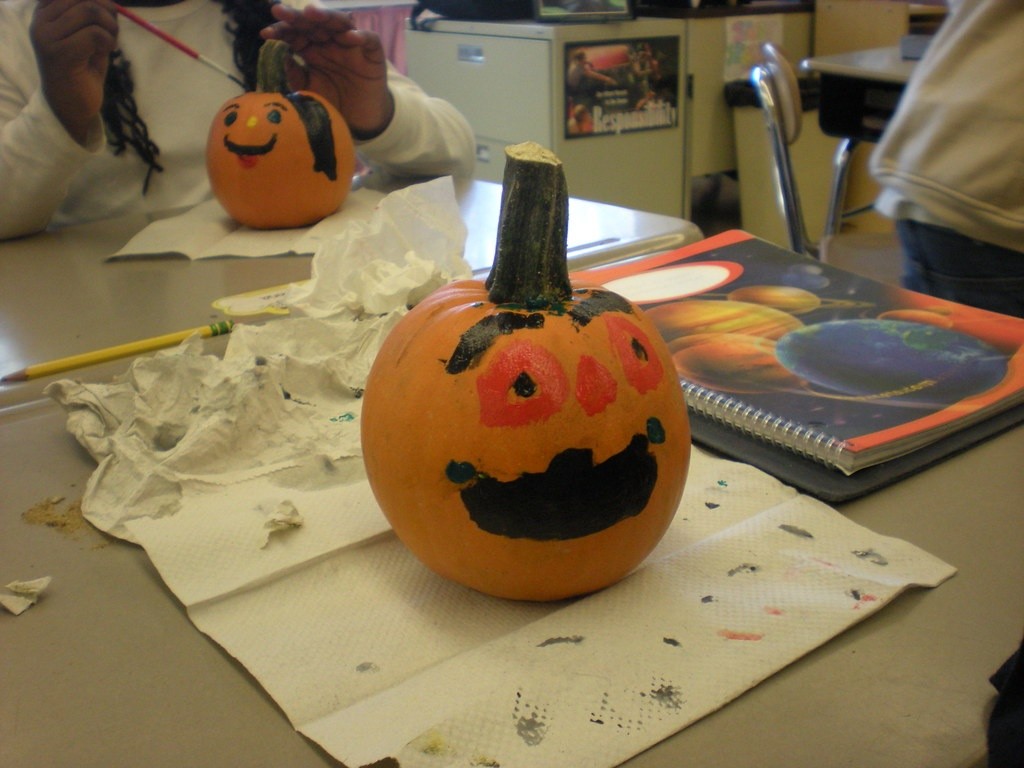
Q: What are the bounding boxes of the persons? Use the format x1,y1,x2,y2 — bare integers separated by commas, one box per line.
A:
0,0,476,241
868,0,1024,317
567,45,670,133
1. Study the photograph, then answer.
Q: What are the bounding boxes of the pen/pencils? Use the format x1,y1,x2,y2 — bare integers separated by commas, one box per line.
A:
0,316,240,386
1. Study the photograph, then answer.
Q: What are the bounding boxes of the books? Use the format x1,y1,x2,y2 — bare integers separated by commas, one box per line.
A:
569,229,1024,475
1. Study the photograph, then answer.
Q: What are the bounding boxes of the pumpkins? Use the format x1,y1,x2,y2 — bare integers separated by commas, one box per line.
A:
205,37,353,228
359,144,691,601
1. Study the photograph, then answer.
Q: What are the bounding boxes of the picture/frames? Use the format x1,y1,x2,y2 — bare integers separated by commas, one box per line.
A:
564,36,680,139
533,0,635,22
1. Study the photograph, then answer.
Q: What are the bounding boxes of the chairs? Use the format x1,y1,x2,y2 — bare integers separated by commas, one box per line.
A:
752,40,905,287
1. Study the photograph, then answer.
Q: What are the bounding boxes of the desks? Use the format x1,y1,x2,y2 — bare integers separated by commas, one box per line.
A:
798,46,924,233
0,175,1023,768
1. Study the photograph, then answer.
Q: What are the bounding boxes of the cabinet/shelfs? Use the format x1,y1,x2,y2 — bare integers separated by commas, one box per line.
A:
403,2,813,222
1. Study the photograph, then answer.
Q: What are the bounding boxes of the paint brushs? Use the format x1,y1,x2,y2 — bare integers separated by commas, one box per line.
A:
111,2,253,92
